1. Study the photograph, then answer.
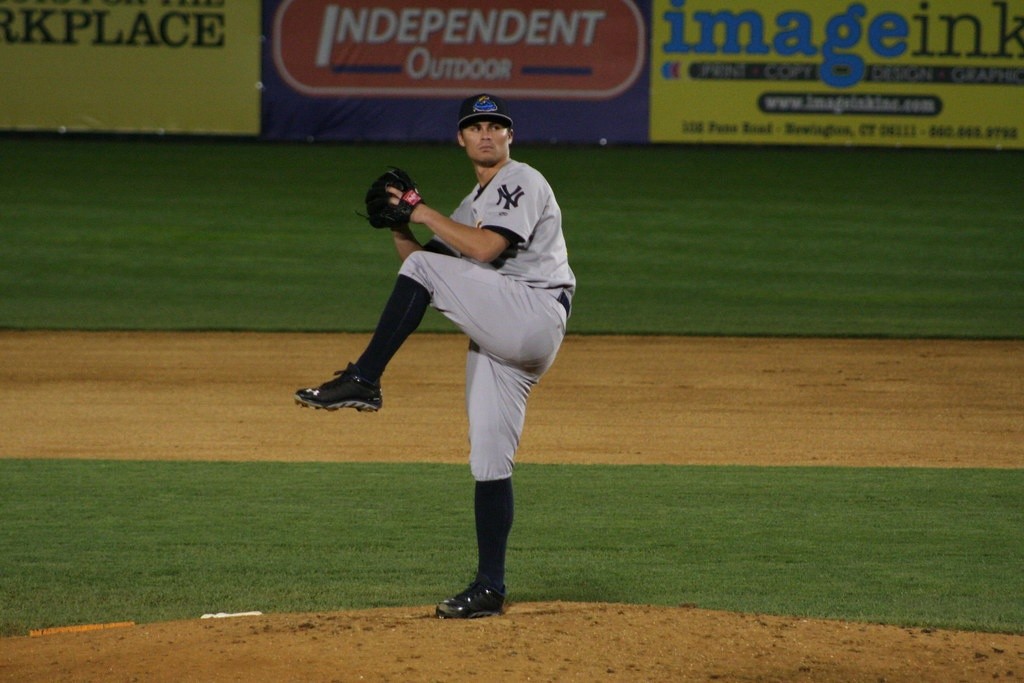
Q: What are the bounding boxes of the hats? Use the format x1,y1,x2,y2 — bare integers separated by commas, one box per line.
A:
457,94,513,127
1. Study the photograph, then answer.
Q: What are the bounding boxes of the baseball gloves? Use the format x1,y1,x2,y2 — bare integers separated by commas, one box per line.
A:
365,168,425,229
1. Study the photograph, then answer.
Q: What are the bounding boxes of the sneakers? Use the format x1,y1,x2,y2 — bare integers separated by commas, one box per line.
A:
294,361,382,412
436,575,506,619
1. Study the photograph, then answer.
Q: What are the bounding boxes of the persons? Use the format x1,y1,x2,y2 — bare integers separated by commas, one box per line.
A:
293,92,576,620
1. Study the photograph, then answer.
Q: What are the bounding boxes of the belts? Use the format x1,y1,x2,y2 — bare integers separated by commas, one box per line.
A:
556,291,570,318
